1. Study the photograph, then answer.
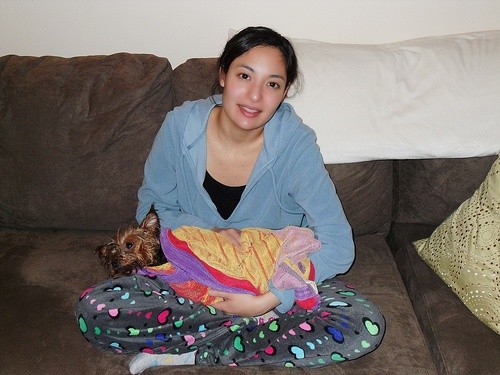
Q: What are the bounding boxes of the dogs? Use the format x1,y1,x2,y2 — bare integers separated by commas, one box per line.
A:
91,202,169,279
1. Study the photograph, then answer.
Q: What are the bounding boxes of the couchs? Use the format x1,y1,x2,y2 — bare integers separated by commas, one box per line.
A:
0,52,500,375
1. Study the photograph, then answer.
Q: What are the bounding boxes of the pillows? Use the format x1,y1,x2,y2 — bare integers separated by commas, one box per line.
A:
282,30,500,165
411,155,500,335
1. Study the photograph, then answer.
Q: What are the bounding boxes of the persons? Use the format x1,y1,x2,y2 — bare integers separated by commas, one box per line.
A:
74,26,386,375
